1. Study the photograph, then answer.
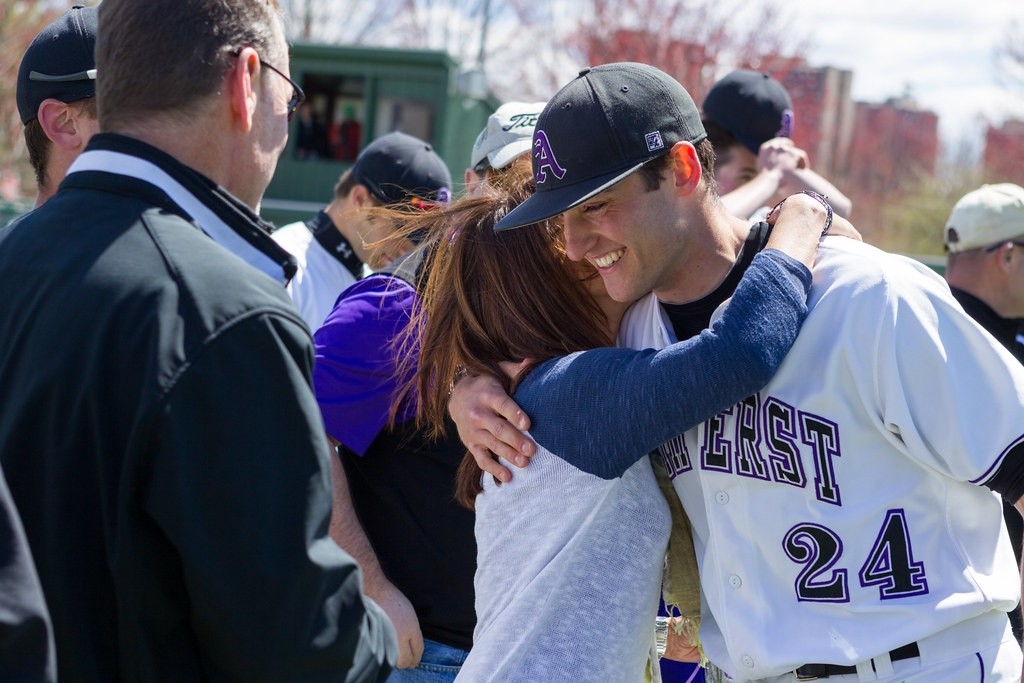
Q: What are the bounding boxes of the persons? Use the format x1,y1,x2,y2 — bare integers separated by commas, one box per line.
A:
3,4,1024,683
493,60,1024,683
2,0,396,683
394,163,868,683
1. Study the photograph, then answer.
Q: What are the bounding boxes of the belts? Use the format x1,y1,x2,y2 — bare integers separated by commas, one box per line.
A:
790,641,920,680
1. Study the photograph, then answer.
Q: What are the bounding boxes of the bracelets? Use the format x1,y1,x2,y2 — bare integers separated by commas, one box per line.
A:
767,188,834,234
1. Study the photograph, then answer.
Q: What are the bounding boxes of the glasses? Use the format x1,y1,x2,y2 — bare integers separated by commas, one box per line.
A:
229,49,306,124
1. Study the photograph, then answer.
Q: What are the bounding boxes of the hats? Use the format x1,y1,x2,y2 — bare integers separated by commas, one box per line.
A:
354,136,452,244
944,183,1024,254
492,62,708,232
16,4,98,125
471,101,548,173
702,70,793,153
